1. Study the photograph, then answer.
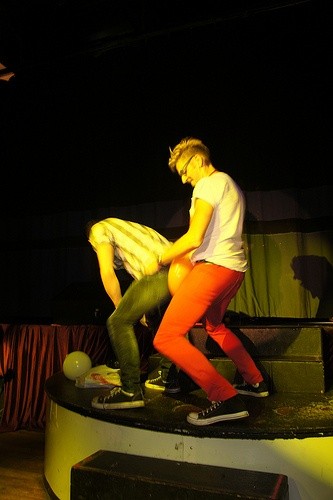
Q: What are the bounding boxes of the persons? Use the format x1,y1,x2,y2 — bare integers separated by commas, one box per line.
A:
78,212,180,409
144,141,270,424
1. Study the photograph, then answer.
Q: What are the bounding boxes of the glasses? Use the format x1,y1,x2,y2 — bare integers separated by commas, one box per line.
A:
179,153,198,177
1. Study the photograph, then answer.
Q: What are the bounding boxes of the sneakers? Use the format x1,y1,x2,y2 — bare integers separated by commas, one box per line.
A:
187,396,250,425
145,376,180,393
232,381,269,397
91,387,145,408
